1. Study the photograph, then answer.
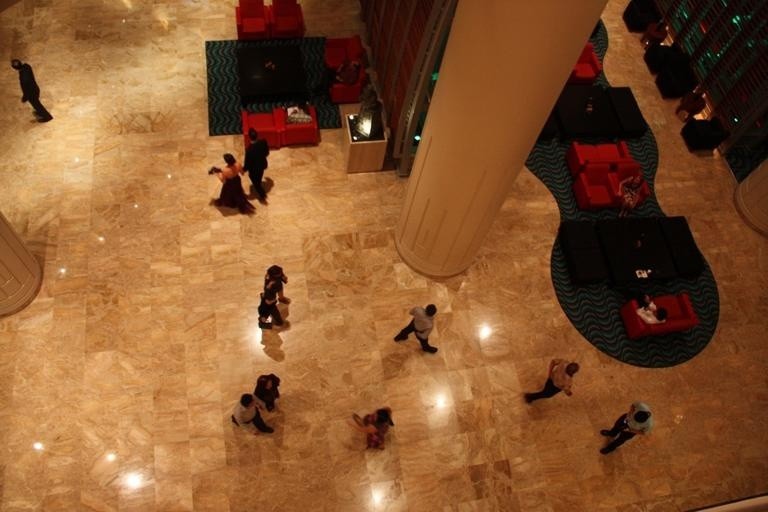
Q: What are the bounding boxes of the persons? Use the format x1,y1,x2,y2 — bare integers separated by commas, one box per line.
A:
346,406,394,450
208,153,257,217
232,393,274,434
599,400,653,455
253,373,281,412
522,358,579,403
11,59,52,123
242,127,269,201
633,289,667,325
618,172,643,217
258,286,283,328
264,265,291,304
394,303,437,352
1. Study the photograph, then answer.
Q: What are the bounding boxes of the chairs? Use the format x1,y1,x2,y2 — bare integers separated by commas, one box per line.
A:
234,0,366,151
624,18,720,154
570,41,602,80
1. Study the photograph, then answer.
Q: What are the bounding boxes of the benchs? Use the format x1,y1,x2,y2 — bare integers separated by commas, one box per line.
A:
567,140,650,210
619,295,700,338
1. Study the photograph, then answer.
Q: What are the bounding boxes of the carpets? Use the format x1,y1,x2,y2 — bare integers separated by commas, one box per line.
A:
525,17,719,367
205,37,342,137
